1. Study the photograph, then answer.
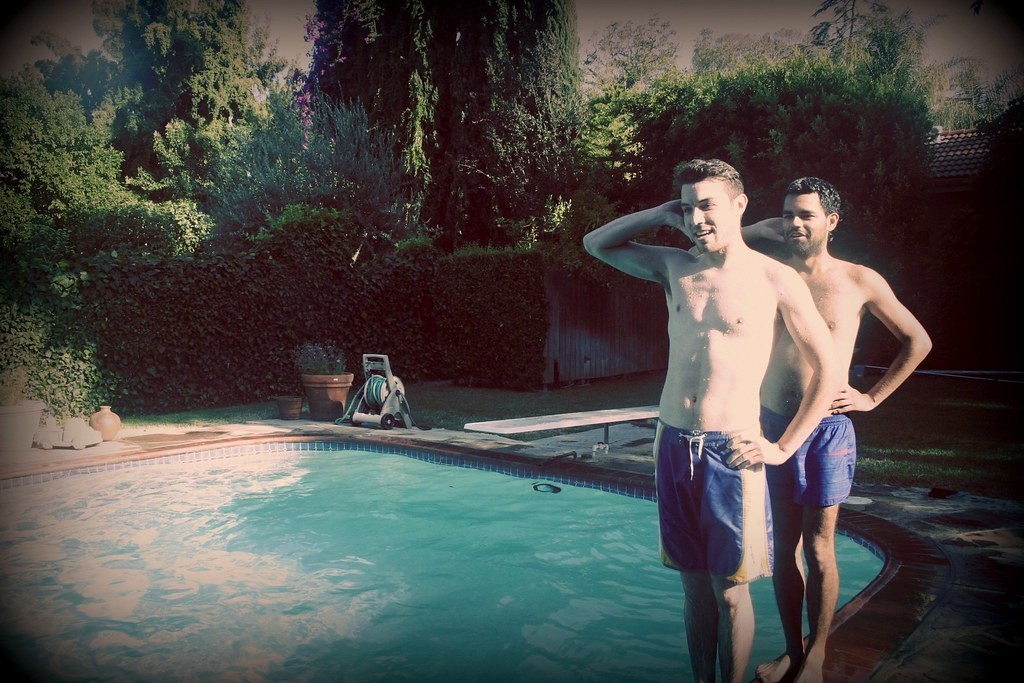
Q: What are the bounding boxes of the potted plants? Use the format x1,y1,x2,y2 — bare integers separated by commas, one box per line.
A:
0,304,45,450
297,340,353,421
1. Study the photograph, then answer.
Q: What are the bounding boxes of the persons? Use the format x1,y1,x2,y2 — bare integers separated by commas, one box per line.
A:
583,159,843,683
690,176,932,683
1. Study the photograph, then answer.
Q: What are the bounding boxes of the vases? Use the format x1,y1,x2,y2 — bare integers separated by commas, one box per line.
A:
90,406,121,441
278,395,302,420
